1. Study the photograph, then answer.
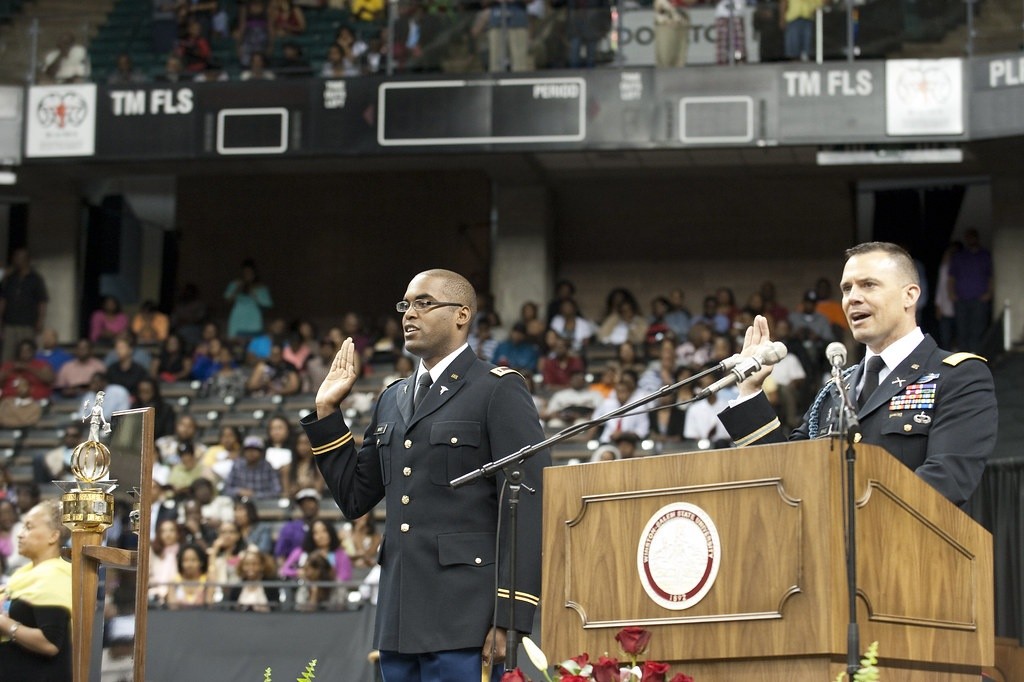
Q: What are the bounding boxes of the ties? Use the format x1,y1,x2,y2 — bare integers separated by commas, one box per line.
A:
857,356,886,411
413,372,434,414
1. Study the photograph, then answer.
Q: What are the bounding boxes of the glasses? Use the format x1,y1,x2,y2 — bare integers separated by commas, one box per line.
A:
396,299,463,313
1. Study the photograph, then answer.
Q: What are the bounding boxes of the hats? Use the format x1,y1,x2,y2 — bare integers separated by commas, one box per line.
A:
243,436,264,450
294,488,321,503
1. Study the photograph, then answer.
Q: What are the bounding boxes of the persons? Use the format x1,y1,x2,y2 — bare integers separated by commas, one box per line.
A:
472,0,825,72
0,233,1004,608
301,268,547,681
0,501,74,680
108,0,442,81
43,33,90,84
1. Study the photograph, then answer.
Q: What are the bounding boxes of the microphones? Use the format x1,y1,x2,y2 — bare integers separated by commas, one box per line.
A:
826,342,847,367
696,341,787,400
